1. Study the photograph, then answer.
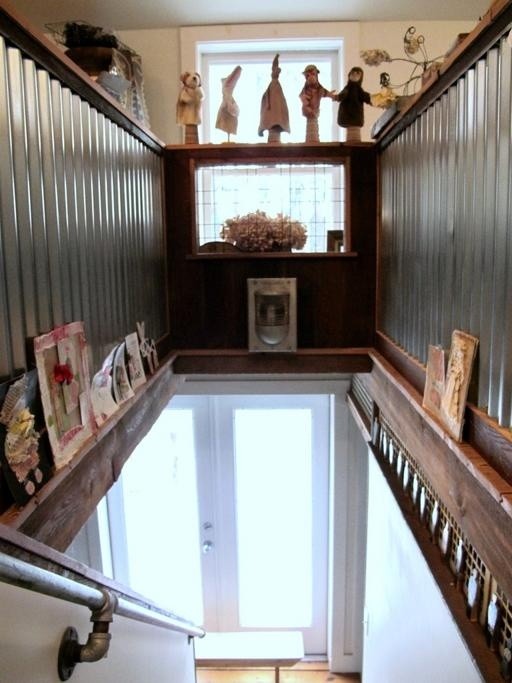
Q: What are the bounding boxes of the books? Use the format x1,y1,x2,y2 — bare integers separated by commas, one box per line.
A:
438,330,478,445
421,343,448,419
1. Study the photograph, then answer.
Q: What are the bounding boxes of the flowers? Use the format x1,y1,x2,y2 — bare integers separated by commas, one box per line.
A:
364,25,447,107
219,210,308,251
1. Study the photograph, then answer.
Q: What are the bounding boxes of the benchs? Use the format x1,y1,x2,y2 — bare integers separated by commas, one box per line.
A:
194,631,305,682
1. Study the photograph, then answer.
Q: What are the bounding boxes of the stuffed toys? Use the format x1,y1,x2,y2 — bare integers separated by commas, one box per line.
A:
257,53,290,146
215,66,242,134
299,64,335,142
332,66,371,142
176,71,205,146
